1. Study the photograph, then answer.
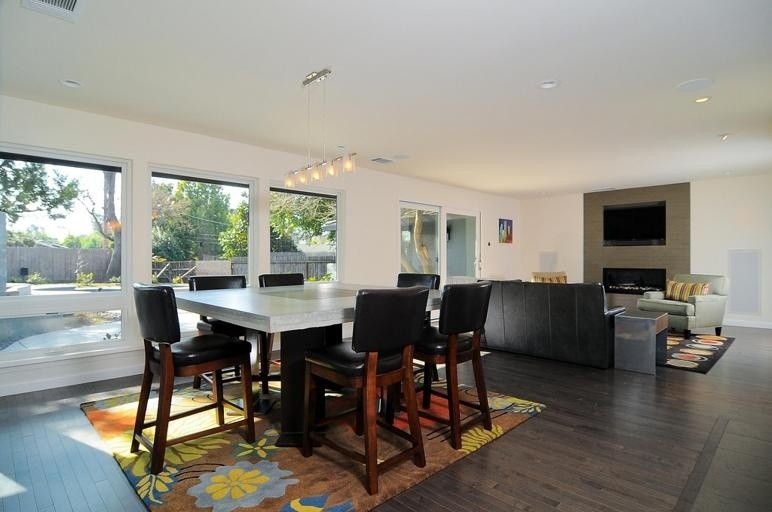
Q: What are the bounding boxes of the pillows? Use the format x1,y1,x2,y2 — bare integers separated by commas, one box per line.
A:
664,278,712,302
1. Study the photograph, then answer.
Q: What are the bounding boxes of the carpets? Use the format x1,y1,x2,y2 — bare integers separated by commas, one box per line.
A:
662,330,736,374
80,358,546,512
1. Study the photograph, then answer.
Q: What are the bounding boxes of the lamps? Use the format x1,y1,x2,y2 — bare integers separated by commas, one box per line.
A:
282,68,358,187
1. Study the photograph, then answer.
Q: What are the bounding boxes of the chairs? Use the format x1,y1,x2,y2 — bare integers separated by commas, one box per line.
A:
383,279,496,451
302,286,431,494
396,272,441,382
531,272,567,283
260,272,304,372
188,273,268,402
635,272,731,338
130,281,255,476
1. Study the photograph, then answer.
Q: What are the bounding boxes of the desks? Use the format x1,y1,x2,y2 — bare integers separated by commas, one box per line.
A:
613,307,669,377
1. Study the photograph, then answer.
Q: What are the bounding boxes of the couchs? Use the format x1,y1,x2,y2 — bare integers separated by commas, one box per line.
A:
472,278,625,370
